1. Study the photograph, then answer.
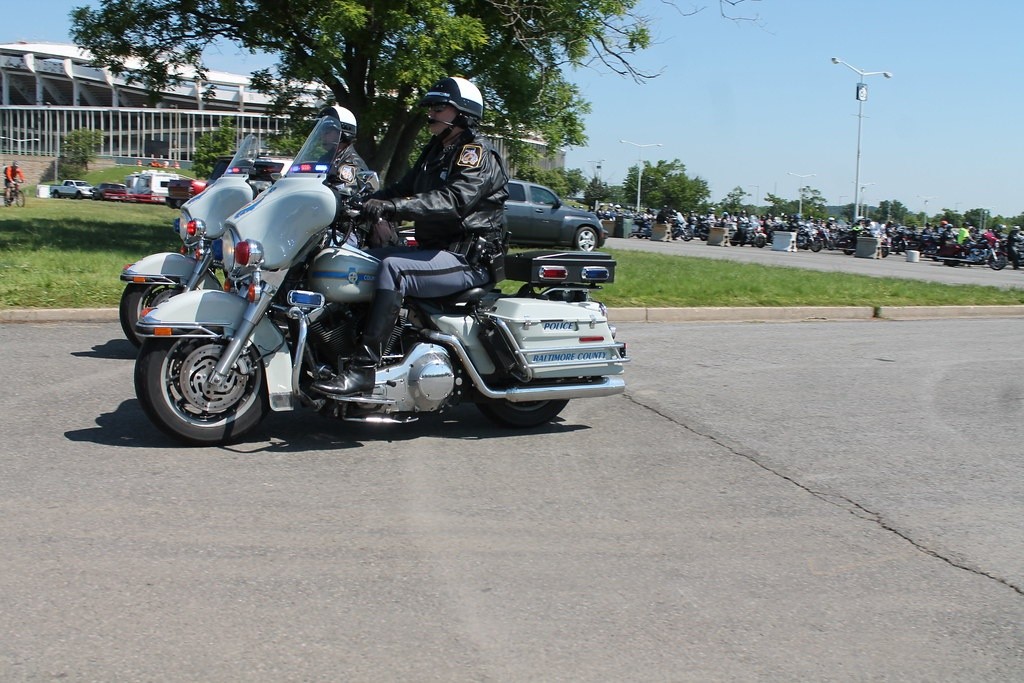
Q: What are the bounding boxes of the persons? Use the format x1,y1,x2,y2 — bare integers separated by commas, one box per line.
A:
312,76,509,399
4,161,25,208
590,202,1024,270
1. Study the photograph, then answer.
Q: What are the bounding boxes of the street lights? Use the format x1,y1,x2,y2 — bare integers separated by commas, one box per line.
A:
786,55,938,226
618,138,665,213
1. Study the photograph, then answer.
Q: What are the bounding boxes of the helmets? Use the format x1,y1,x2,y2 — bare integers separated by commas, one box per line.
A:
316,106,357,142
567,197,1023,241
418,76,483,121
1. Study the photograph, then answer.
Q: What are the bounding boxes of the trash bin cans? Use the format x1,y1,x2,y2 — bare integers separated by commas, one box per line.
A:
613,215,633,238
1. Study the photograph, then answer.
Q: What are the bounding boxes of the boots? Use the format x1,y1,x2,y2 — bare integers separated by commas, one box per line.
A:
309,290,404,399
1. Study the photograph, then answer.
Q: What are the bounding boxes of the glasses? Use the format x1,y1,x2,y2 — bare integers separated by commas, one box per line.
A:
428,103,448,111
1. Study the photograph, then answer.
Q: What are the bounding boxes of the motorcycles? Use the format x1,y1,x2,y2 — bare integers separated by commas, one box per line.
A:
128,115,628,444
589,201,1024,274
114,133,417,349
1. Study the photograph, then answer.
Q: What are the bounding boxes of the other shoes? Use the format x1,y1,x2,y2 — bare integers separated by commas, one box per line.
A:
5,199,11,205
15,193,20,198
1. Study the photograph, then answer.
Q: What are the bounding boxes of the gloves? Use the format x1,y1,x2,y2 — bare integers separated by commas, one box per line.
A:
360,198,396,222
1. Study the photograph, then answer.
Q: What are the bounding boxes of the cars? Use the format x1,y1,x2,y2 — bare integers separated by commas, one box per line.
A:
494,179,604,252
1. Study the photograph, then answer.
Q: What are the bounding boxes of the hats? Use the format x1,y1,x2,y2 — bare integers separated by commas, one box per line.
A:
14,160,19,168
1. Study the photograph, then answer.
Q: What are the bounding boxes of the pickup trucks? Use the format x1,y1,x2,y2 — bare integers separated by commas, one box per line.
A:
48,154,294,212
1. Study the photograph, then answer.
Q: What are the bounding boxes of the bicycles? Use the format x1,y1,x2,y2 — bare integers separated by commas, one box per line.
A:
2,179,26,208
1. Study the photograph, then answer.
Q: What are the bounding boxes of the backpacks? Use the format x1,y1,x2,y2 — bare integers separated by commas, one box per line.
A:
3,166,8,176
